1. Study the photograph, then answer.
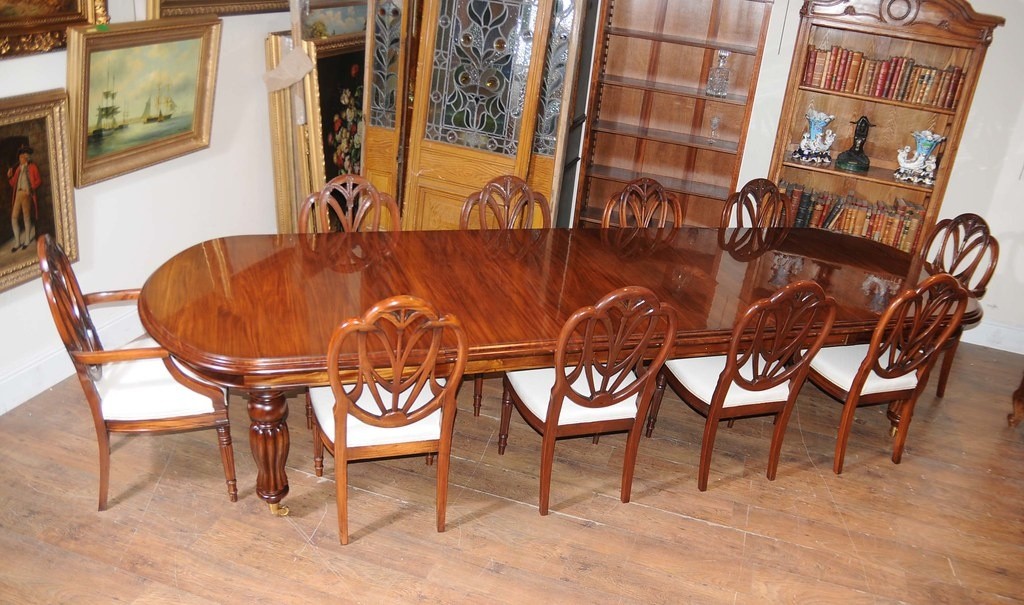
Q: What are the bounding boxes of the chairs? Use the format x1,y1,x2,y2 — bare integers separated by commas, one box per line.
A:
866,214,998,396
308,294,468,546
497,286,679,515
296,174,400,235
646,280,840,491
719,179,790,230
772,274,965,474
37,233,239,511
459,175,551,416
601,177,683,229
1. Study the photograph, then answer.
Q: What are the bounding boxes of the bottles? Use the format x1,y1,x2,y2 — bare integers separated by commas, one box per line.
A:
705,49,731,98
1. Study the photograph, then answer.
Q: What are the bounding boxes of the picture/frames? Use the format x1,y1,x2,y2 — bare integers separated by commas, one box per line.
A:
0,87,80,295
265,0,366,234
145,0,290,20
66,12,223,190
0,0,111,58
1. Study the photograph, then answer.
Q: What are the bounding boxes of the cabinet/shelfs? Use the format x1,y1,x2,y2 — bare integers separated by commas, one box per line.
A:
756,0,1007,255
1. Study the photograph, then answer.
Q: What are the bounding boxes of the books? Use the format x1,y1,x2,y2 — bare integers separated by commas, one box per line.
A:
803,43,966,110
772,181,927,253
